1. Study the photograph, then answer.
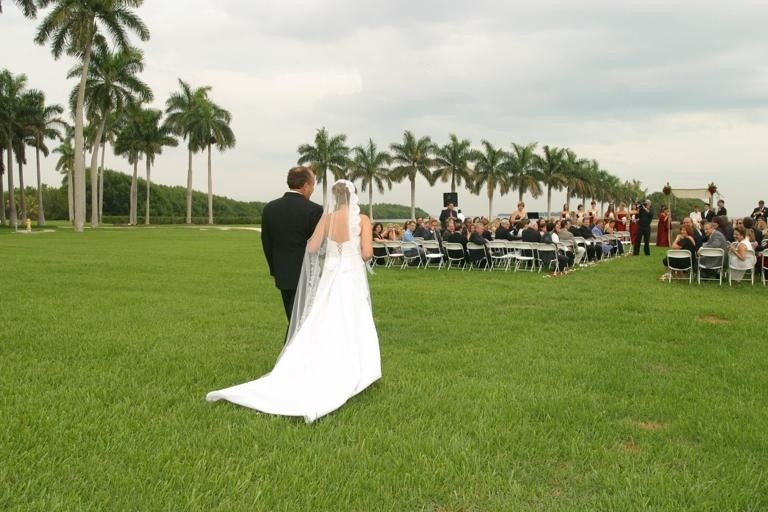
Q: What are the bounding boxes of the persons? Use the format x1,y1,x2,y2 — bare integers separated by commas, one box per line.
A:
204,178,383,423
261,166,324,346
370,200,653,272
657,201,767,280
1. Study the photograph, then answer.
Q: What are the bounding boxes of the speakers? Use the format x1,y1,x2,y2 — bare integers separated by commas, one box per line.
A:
443,192,458,207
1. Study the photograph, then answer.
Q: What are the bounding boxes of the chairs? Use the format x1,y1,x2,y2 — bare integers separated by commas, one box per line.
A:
666,240,768,286
369,230,633,276
